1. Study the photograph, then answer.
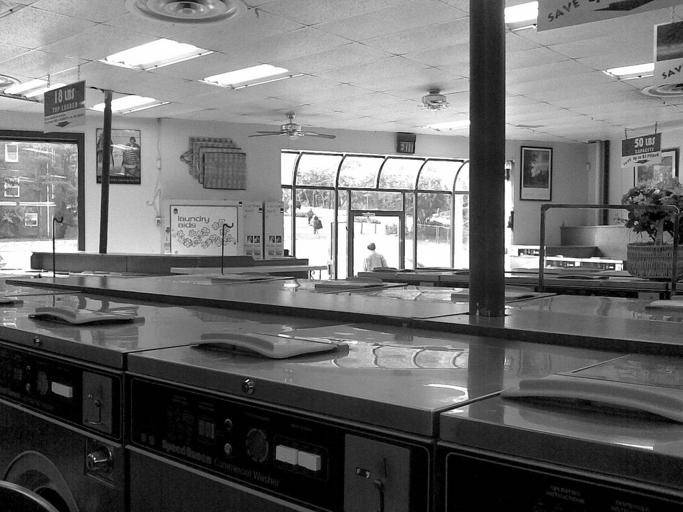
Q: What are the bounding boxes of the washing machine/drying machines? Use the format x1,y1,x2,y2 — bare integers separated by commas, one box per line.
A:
0,266,683,512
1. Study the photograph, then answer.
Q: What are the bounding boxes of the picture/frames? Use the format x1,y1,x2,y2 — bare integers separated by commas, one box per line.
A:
520,146,552,201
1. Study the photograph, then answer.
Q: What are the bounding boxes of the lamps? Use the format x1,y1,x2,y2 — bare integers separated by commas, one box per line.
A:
417,88,449,111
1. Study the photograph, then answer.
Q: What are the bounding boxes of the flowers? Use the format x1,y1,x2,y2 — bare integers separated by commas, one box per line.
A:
622,176,682,245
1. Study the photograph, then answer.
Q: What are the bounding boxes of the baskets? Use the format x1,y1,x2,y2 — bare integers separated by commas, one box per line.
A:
624,241,683,281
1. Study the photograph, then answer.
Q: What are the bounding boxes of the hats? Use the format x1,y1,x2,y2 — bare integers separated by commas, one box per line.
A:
367,243,376,250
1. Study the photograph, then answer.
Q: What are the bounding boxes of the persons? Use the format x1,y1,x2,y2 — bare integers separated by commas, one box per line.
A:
362,241,389,272
98,131,140,176
306,208,323,235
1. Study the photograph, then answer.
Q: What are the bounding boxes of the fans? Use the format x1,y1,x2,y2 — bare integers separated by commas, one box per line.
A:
248,113,336,139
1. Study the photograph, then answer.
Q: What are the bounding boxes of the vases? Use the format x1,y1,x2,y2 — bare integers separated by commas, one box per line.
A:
628,243,683,278
